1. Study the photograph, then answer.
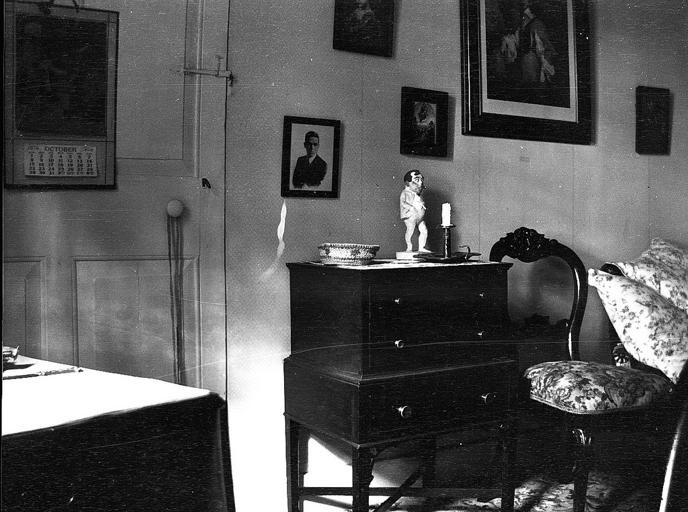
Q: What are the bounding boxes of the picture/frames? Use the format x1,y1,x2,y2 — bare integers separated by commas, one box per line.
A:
279,0,449,199
460,1,592,146
635,85,670,154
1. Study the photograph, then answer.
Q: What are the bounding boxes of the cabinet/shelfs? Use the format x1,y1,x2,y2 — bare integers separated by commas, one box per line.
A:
284,261,515,512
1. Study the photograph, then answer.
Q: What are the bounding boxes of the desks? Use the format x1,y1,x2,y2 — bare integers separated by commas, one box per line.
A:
2,355,236,512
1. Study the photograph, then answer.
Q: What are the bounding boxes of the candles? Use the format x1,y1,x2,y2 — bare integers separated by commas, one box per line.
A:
441,203,451,226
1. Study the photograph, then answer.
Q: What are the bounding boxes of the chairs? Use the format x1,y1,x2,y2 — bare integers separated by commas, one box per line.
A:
488,226,684,512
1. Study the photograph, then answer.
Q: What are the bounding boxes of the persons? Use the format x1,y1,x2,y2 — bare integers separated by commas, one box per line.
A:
400,170,431,253
498,4,555,84
293,131,328,188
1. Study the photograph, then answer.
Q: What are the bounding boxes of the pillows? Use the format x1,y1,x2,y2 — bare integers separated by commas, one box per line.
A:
588,237,687,386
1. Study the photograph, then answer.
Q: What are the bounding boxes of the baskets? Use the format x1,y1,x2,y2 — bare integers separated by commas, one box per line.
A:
317,243,380,266
